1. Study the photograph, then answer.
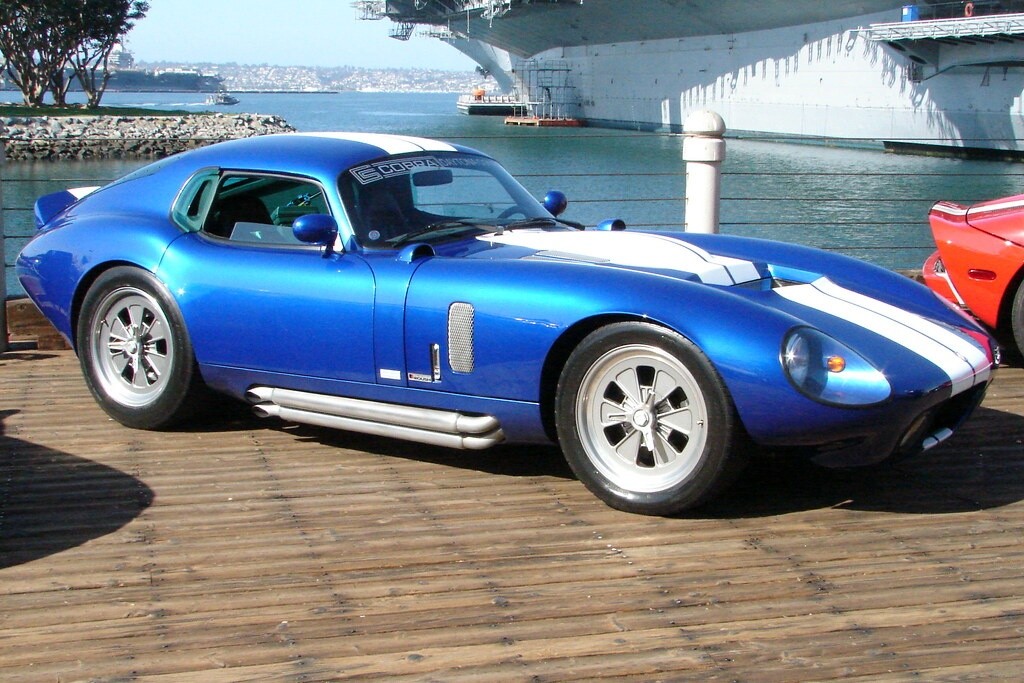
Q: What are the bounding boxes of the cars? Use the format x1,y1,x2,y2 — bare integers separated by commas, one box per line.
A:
922,194,1024,364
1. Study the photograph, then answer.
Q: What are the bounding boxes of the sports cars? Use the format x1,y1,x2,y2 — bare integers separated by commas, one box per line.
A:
15,130,1002,518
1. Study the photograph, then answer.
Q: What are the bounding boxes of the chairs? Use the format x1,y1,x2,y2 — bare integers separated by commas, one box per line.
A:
210,197,288,244
344,179,422,247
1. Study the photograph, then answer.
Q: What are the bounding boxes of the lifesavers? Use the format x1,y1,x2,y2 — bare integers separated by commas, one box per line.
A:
964,3,975,17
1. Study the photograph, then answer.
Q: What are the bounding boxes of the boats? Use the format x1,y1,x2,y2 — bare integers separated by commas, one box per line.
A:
213,92,240,105
